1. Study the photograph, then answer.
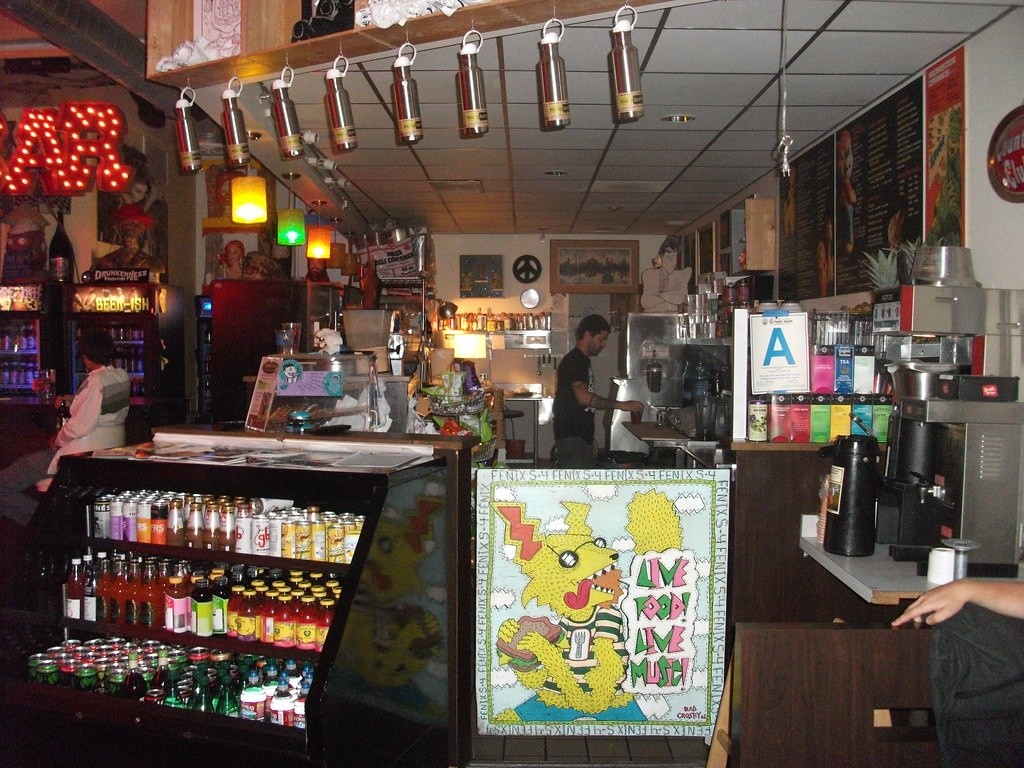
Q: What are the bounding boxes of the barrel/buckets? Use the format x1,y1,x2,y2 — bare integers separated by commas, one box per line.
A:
506,439,525,459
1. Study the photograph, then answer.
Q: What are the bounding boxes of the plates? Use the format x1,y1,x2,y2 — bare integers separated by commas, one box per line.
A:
513,392,532,397
598,450,649,464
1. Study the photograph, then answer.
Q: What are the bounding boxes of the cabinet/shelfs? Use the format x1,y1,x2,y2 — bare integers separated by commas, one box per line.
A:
376,265,434,393
729,197,777,275
1,442,451,768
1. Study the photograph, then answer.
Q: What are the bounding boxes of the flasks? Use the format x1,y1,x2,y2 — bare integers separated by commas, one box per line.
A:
817,414,886,557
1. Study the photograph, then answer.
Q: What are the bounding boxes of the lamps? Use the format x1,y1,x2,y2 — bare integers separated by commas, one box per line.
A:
278,173,307,246
436,299,459,319
231,132,268,224
302,131,350,189
306,199,358,276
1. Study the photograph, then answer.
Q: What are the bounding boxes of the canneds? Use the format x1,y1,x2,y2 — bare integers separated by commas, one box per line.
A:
23,637,285,708
235,507,366,565
91,488,208,545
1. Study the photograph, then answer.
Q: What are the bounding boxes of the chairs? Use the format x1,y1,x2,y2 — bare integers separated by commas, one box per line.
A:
930,601,1024,768
504,405,524,441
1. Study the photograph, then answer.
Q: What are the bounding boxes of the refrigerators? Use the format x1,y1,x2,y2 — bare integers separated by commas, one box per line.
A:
56,282,184,446
0,278,61,394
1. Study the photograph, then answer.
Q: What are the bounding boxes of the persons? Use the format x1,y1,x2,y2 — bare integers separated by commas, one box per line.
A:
891,578,1024,627
0,330,129,498
215,239,245,278
552,314,644,469
94,218,165,272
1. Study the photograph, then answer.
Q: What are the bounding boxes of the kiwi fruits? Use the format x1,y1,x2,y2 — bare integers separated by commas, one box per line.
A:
840,301,874,312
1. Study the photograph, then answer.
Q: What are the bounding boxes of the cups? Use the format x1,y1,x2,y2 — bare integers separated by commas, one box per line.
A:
816,473,834,543
275,322,302,356
927,547,955,586
631,411,642,424
694,397,732,442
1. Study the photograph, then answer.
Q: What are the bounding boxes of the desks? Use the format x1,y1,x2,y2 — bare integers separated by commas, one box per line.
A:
493,382,542,469
622,421,690,468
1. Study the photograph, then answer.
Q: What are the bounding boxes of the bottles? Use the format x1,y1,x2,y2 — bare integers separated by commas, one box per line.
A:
49,212,75,285
57,400,70,433
75,321,145,397
438,308,553,332
204,319,214,416
19,487,343,731
40,367,53,405
175,5,644,173
0,316,40,395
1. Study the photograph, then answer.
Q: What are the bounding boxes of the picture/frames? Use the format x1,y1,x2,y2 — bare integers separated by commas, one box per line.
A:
549,238,640,294
677,208,730,295
460,254,505,298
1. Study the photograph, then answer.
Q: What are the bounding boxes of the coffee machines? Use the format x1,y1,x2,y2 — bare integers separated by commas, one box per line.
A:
875,399,1024,582
